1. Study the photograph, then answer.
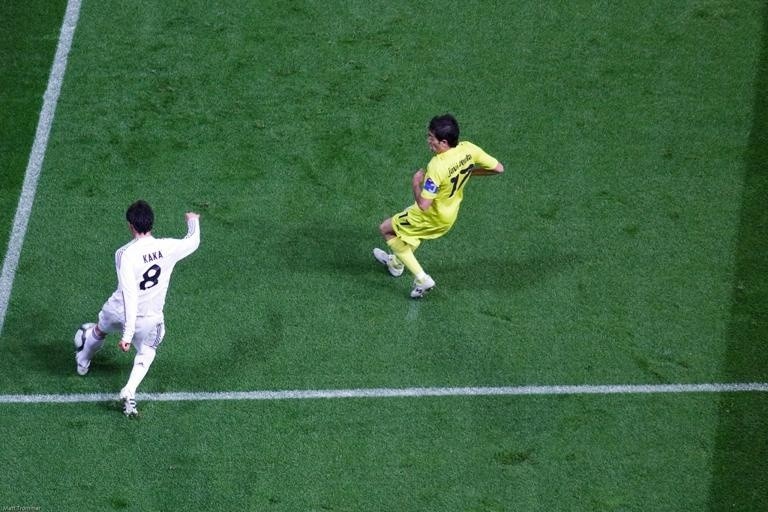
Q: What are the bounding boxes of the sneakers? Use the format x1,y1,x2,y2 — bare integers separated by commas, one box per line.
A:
373,248,404,277
409,276,436,299
75,351,92,375
120,387,138,418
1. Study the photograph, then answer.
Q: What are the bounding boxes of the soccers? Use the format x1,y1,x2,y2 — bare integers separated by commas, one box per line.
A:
74,322,103,355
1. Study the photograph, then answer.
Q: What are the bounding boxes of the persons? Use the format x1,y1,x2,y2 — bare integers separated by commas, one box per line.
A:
72,199,202,418
370,113,504,299
